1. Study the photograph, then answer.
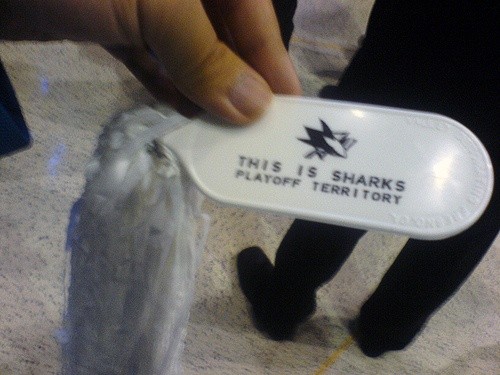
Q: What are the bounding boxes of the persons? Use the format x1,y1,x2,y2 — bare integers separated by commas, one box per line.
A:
230,0,500,358
0,0,306,157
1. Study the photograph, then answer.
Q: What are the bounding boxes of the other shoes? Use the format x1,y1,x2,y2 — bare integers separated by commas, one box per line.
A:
238,246,292,339
356,319,388,358
